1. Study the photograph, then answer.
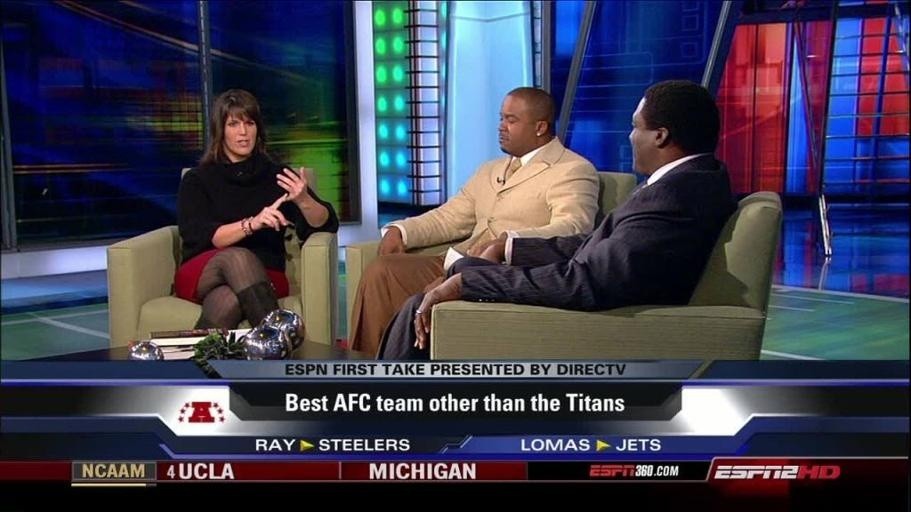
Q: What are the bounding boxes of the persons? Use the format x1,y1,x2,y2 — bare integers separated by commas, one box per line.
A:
173,87,341,330
345,85,602,361
375,78,733,362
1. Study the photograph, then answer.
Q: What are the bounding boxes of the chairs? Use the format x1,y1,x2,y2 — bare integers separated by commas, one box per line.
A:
344,171,640,354
431,190,784,362
107,167,339,360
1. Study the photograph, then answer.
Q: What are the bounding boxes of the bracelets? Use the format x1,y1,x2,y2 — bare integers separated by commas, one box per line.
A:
241,217,253,235
248,215,259,234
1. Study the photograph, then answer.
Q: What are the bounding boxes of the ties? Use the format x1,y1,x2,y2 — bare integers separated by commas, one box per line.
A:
508,158,522,178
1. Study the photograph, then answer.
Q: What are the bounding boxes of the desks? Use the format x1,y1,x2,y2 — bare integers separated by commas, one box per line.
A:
16,330,401,360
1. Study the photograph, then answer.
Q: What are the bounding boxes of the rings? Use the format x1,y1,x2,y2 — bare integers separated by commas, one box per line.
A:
416,309,423,314
